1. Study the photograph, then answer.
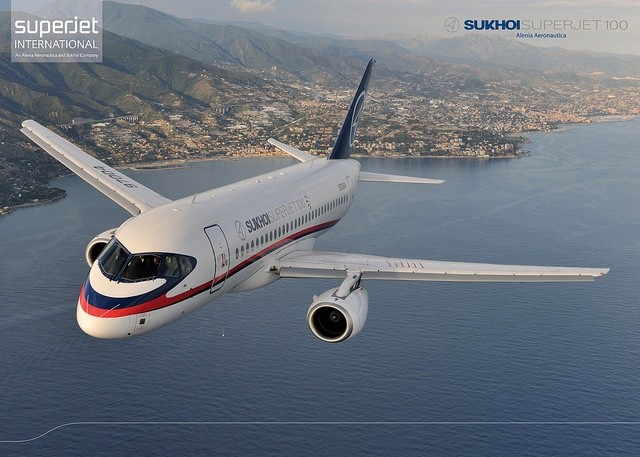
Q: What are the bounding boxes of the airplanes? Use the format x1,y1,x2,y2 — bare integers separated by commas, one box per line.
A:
19,57,611,344
